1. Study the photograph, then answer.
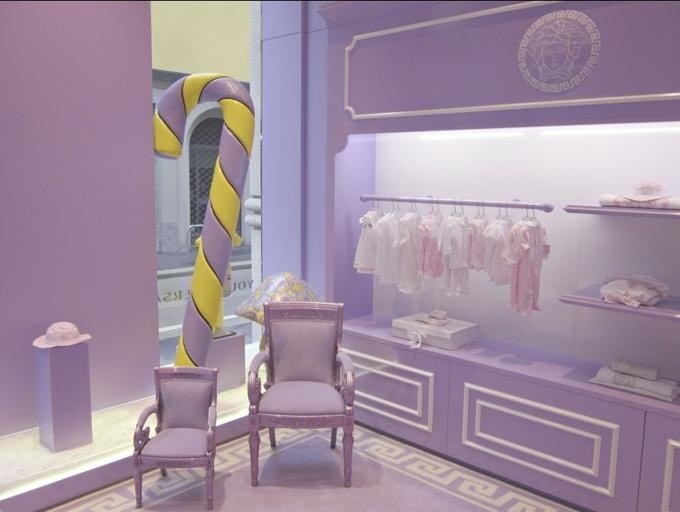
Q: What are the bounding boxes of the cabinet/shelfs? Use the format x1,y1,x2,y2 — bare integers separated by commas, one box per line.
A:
558,205,680,321
338,314,680,512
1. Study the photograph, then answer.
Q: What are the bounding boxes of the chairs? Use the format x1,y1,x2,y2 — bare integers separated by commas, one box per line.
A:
132,362,220,510
248,301,357,491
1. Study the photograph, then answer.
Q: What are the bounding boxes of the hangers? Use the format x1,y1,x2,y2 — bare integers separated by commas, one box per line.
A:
361,195,544,239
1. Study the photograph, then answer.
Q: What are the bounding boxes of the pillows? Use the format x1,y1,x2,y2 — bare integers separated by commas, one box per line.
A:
235,271,331,324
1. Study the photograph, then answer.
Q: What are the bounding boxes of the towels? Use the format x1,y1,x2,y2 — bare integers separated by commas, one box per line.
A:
588,360,679,405
